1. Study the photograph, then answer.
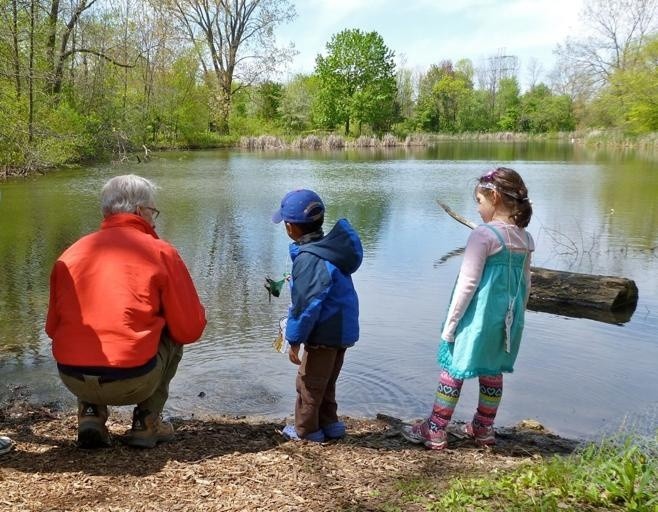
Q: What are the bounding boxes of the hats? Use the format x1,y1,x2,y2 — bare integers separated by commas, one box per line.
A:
272,188,325,225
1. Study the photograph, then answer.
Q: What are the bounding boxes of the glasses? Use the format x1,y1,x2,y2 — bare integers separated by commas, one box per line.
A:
144,206,160,218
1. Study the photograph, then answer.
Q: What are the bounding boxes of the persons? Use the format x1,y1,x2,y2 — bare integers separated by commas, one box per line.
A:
268,188,364,445
397,164,537,451
43,172,209,450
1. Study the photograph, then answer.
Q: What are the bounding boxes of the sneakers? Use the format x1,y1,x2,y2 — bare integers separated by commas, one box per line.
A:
283,425,324,442
400,421,448,450
323,422,345,438
464,418,496,445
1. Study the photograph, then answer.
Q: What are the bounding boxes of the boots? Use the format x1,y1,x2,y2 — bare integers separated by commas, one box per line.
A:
77,400,112,449
127,406,174,448
